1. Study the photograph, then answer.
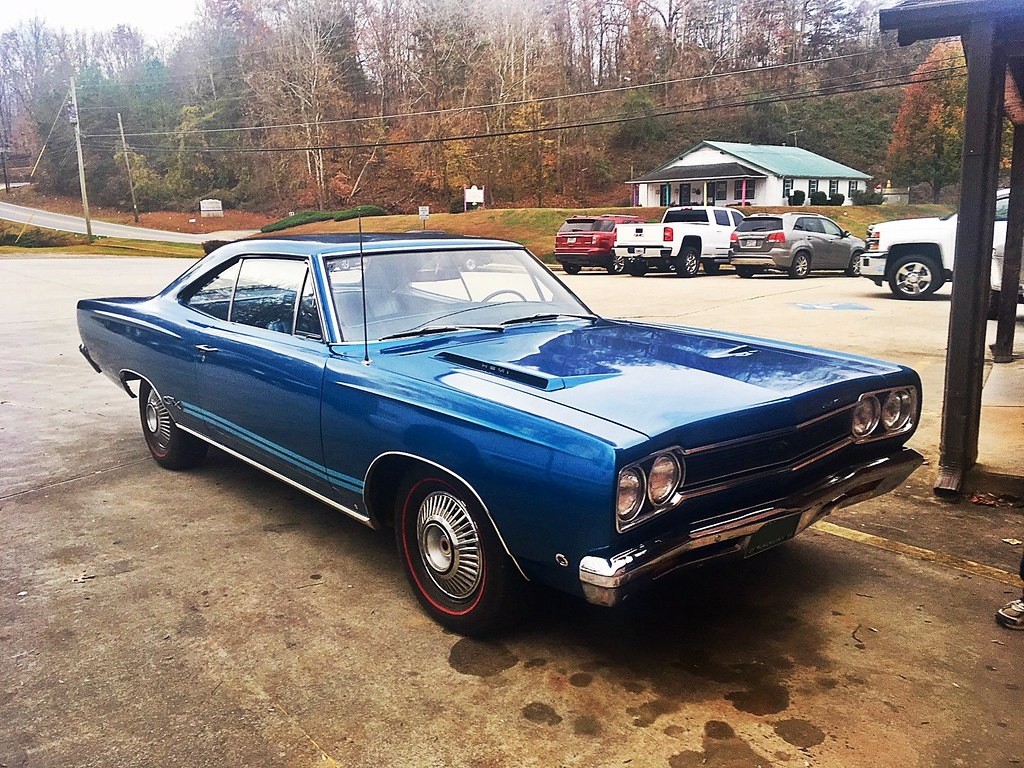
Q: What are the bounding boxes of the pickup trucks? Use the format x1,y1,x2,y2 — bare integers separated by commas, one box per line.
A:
613,205,759,279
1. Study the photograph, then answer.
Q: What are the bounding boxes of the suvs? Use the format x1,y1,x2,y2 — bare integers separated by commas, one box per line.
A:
859,189,1012,298
728,211,865,279
552,213,677,275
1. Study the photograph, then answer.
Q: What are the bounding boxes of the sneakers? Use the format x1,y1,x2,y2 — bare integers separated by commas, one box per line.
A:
994,598,1024,631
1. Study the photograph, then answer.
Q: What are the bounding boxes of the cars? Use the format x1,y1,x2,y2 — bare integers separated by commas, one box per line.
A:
987,242,1024,320
75,231,924,638
